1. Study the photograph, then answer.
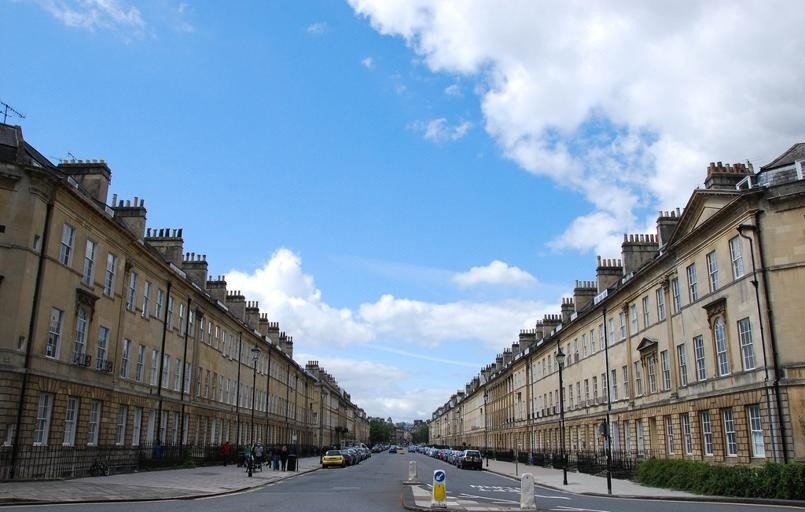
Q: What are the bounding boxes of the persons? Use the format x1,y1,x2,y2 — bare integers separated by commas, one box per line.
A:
220,440,288,472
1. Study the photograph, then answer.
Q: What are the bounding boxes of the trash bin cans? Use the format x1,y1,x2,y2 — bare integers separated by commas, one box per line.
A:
288,455,296,471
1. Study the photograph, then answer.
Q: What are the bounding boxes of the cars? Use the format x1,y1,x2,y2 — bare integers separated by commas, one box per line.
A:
409,444,483,470
322,442,403,469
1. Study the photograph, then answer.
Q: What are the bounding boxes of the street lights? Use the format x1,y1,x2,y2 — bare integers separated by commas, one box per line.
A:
552,340,570,485
249,343,260,448
483,392,489,467
